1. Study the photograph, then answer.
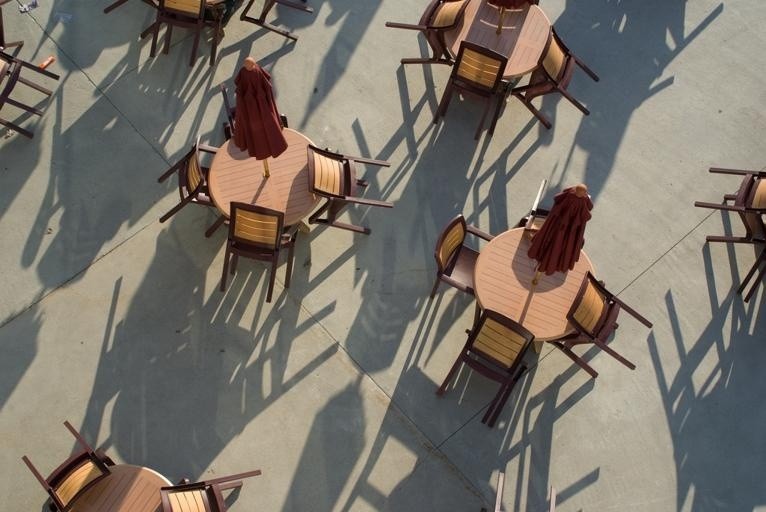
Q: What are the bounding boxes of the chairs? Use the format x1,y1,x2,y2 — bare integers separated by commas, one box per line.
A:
510,24,601,132
102,1,159,39
694,167,765,305
146,0,227,68
434,307,536,429
429,213,496,325
0,49,62,143
154,466,261,512
546,268,653,381
306,142,395,236
384,0,468,67
214,199,297,305
431,39,510,141
217,81,290,142
237,0,319,44
18,417,115,506
519,178,593,249
156,134,225,238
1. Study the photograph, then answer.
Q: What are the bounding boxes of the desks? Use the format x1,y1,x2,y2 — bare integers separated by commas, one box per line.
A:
58,463,173,512
441,1,552,80
207,126,324,229
470,225,595,344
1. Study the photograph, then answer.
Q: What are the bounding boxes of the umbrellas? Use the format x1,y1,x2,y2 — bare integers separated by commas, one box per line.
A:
524,182,595,288
232,58,290,181
